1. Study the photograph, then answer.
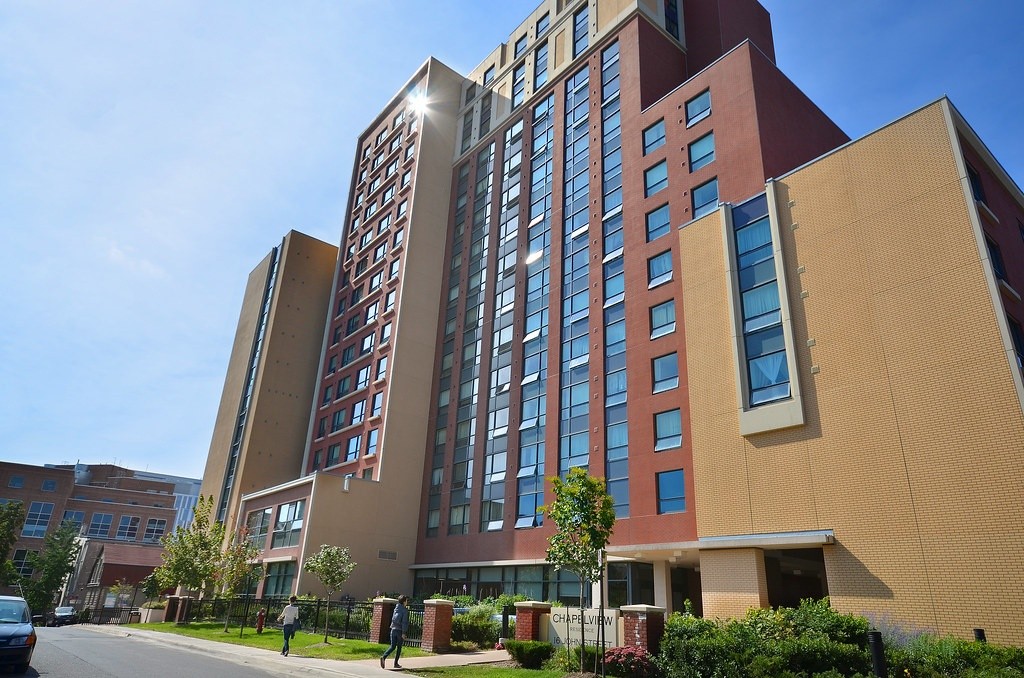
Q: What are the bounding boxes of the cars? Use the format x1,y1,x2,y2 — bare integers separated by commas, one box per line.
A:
53,603,78,627
0,596,43,674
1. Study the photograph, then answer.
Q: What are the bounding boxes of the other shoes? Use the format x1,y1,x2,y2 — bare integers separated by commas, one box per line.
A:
394,664,402,668
380,656,385,669
280,651,284,655
284,650,288,657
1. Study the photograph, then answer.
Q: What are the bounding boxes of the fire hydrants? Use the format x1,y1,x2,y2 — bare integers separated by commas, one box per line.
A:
256,608,265,634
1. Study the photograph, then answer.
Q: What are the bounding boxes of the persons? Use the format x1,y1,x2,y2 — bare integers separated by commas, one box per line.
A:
380,595,408,669
276,595,299,656
